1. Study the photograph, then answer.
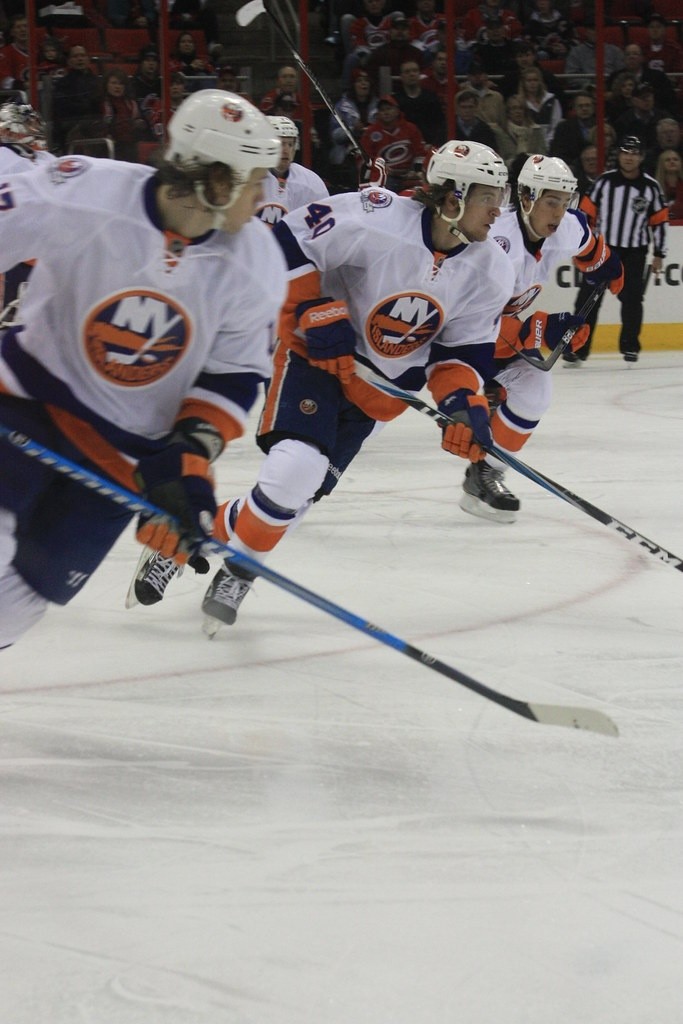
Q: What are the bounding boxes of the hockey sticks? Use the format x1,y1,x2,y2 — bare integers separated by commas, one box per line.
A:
0,427,623,740
235,0,374,169
293,322,683,574
498,279,609,371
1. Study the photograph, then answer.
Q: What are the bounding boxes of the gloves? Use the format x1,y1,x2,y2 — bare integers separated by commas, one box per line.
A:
573,235,624,295
295,296,359,385
132,418,226,566
437,388,493,464
520,311,590,354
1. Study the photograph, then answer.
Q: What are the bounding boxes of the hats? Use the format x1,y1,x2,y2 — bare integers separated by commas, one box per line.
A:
647,13,667,26
632,81,656,96
376,96,399,107
143,42,158,56
277,92,301,107
352,68,368,79
388,11,407,21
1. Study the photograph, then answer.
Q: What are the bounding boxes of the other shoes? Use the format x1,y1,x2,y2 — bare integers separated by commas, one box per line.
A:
623,352,639,369
562,353,587,368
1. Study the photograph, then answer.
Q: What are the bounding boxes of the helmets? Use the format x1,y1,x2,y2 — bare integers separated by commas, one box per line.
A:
163,87,282,210
267,116,299,149
618,134,647,154
518,154,578,214
426,140,509,223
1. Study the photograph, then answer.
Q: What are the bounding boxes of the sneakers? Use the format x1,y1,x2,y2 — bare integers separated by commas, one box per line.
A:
459,458,519,524
201,559,256,640
125,545,185,610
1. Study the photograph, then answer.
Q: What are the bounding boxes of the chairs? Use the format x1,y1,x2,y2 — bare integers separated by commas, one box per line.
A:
35,0,683,226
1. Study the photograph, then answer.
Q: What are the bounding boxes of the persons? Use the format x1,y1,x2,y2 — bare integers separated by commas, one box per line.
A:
124,139,517,640
0,0,683,366
0,90,289,650
459,154,624,525
